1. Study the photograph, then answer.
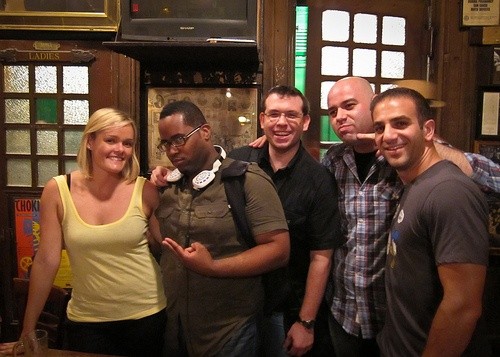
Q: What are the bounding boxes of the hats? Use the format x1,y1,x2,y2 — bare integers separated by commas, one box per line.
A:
397,78,447,107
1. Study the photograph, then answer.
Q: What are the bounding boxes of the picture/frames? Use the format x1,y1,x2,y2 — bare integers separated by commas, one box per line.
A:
0,0,121,32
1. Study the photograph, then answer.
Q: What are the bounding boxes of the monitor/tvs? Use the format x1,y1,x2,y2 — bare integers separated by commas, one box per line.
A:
120,0,257,43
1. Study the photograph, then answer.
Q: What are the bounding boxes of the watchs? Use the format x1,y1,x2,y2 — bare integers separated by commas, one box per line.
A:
297,315,315,329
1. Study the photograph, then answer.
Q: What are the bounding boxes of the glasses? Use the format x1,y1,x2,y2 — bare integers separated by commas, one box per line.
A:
264,110,304,120
157,126,202,153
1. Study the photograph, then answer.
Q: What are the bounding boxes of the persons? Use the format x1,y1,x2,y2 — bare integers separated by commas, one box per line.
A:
248,77,500,357
370,86,491,357
155,100,291,357
150,85,344,357
12,108,164,357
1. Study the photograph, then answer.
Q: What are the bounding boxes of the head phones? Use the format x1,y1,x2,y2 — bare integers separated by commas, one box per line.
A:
166,144,227,191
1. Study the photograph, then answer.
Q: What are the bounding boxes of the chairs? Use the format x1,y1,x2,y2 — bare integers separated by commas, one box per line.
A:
13,277,69,349
